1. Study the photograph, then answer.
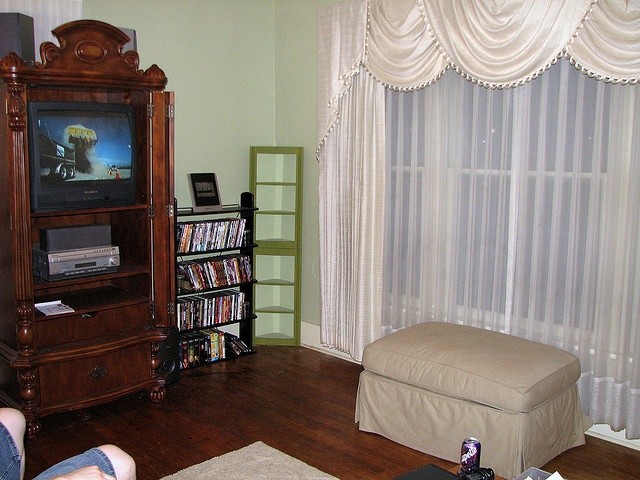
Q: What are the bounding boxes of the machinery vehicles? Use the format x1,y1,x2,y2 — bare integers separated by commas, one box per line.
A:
69,128,96,173
39,125,75,180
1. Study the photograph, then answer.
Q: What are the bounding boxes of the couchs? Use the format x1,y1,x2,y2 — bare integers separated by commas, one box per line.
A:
354,321,592,479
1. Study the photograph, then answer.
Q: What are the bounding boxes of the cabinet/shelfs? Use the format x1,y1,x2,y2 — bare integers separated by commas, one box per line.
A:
0,20,178,440
173,192,258,370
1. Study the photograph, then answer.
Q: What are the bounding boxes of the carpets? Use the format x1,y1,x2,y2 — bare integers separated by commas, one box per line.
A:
157,441,343,480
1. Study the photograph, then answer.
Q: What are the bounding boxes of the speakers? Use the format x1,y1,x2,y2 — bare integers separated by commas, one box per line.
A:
156,326,179,386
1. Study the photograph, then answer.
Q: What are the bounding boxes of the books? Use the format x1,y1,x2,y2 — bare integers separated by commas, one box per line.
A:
173,257,253,297
188,173,223,214
180,290,250,331
177,220,247,253
178,328,255,370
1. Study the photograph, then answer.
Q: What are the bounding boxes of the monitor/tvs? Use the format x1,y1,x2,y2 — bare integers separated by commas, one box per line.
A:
29,102,144,212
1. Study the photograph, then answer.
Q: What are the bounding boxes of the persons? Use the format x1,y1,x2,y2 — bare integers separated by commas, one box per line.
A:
0,406,137,480
